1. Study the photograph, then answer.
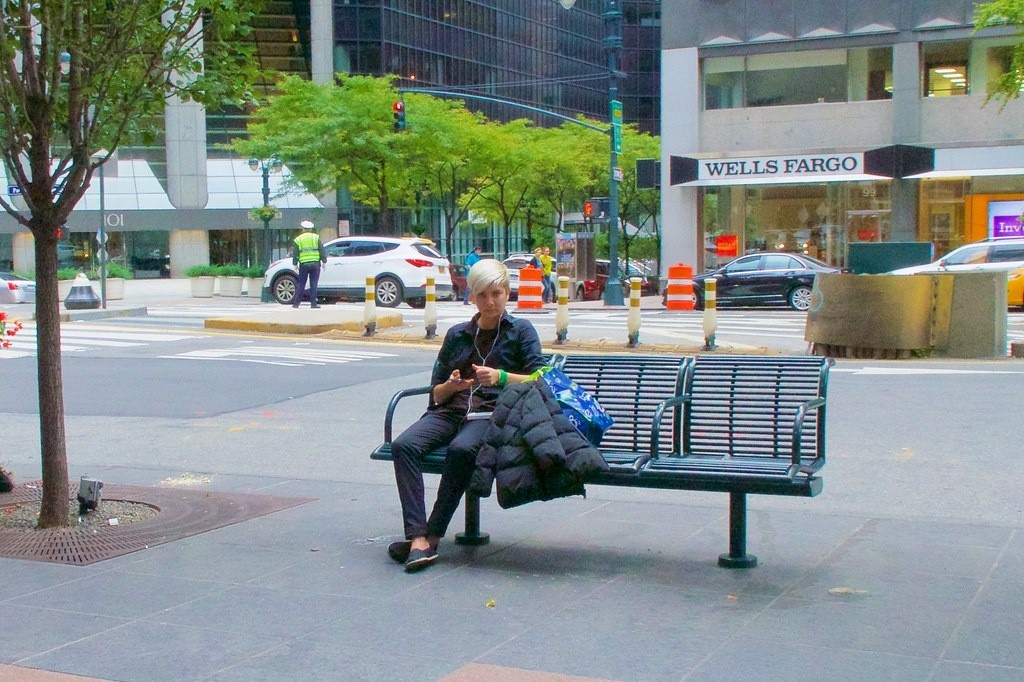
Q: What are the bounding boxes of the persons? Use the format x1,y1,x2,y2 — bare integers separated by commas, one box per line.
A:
463,246,482,304
530,247,553,303
292,221,327,308
556,239,575,277
387,259,547,572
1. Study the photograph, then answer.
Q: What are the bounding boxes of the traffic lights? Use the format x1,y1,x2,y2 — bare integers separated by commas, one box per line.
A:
584,199,601,218
393,101,406,131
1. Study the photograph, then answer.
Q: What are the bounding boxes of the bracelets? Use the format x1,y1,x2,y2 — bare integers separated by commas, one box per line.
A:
498,369,507,386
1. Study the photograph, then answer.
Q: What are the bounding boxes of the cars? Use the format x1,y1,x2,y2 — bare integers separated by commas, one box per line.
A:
662,253,840,311
449,264,467,301
57,226,69,239
576,259,652,301
0,272,36,304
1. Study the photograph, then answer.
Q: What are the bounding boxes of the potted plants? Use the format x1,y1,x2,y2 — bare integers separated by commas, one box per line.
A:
216,262,248,298
245,263,265,298
59,267,80,302
99,260,132,300
186,260,218,298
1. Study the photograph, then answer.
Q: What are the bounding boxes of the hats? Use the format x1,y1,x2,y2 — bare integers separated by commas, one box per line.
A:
301,220,314,229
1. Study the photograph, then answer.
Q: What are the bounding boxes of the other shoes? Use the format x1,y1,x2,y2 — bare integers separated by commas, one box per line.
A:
386,541,439,571
311,306,321,308
292,306,298,308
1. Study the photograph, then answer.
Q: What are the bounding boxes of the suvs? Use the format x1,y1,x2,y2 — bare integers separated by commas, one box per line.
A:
503,253,559,304
883,236,1024,311
265,237,453,309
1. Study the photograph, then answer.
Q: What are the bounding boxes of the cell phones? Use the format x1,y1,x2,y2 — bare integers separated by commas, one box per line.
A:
451,378,466,382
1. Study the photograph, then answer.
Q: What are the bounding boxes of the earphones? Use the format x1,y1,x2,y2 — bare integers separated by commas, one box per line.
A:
506,295,509,301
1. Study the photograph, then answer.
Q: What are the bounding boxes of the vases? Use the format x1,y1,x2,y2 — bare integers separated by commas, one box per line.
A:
262,215,272,221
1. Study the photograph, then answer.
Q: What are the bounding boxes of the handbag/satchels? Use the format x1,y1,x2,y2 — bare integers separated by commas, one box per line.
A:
521,365,615,447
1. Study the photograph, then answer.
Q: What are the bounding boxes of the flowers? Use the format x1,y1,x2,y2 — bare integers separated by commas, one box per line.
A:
248,202,282,221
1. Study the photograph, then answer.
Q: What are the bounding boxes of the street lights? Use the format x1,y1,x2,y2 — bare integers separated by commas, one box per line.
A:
91,148,112,309
248,150,284,273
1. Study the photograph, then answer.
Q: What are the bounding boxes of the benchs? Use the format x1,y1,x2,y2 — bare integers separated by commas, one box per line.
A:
369,353,836,568
